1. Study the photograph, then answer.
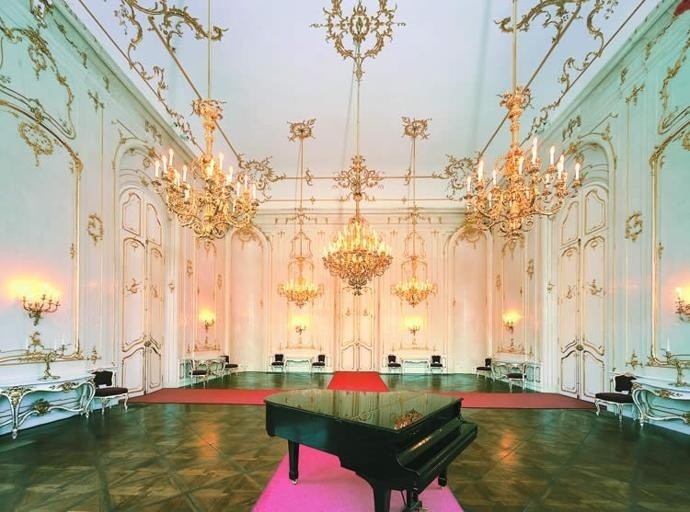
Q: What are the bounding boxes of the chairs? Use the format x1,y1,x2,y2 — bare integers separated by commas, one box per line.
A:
86,368,131,416
506,362,528,392
386,353,402,374
430,354,445,374
220,354,243,380
592,370,640,422
188,358,210,391
311,354,328,373
475,356,494,382
269,351,287,373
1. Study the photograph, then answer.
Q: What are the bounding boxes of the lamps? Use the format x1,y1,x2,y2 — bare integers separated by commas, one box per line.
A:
147,0,261,248
459,0,585,244
0,272,70,324
393,115,439,307
399,315,428,338
310,0,410,298
502,309,523,335
674,284,690,321
197,308,217,331
287,314,316,338
276,119,326,309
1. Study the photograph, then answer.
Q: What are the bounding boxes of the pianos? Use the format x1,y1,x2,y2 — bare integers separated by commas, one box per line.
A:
264,391,477,511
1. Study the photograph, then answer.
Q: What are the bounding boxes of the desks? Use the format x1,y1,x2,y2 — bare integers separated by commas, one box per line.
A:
400,357,432,375
629,377,690,428
197,356,226,385
0,376,96,437
492,360,526,385
284,355,315,372
